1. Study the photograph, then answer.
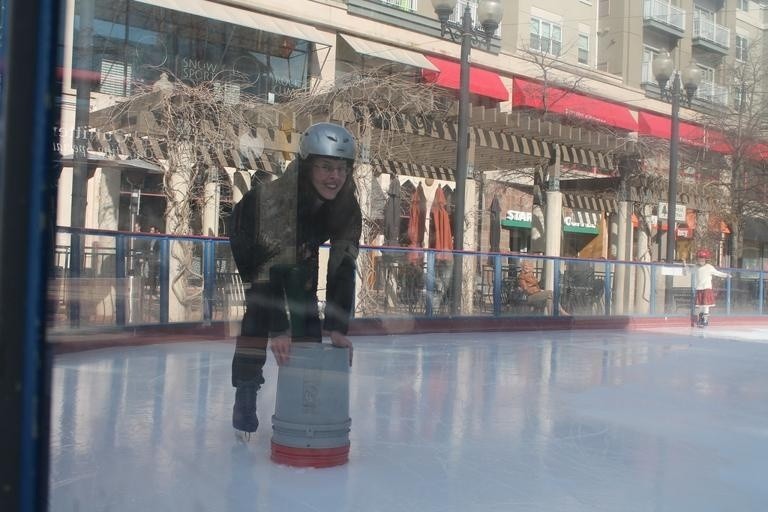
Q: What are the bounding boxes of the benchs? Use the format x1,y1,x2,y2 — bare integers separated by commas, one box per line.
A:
213,273,248,320
504,270,605,317
388,263,484,316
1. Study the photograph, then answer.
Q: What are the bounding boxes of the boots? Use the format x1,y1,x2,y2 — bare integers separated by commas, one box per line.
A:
702,314,709,326
694,315,700,324
230,381,262,435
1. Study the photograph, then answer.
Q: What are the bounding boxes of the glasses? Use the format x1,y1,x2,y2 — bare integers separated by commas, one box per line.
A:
309,161,353,176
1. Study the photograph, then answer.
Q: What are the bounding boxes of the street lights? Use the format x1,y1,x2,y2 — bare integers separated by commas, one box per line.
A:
430,0,504,317
652,47,702,317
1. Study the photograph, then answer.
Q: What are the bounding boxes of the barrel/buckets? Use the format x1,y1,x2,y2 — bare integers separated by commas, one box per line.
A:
272,340,351,466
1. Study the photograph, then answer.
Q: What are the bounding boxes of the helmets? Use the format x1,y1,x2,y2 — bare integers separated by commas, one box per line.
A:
299,121,359,166
695,249,708,259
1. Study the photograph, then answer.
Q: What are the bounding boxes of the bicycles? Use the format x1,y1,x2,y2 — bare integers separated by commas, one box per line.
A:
488,270,604,314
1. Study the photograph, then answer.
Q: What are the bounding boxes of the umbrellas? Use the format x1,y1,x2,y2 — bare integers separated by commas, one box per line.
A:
377,176,407,259
402,178,427,268
487,194,503,268
429,181,454,268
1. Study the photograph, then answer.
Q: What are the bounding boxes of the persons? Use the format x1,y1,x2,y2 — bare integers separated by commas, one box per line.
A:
518,260,574,317
132,221,147,276
226,121,364,435
682,250,733,328
140,225,162,295
